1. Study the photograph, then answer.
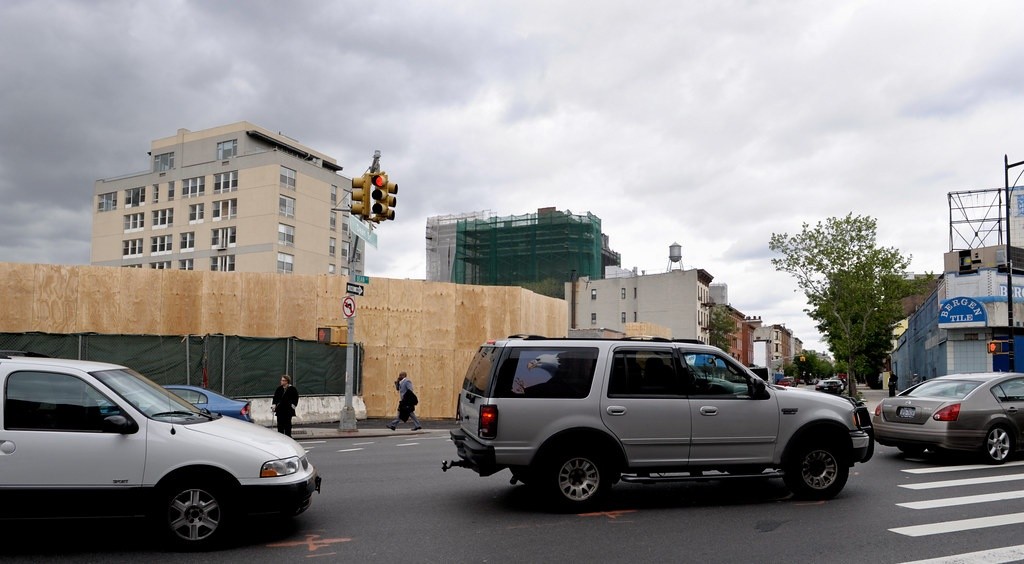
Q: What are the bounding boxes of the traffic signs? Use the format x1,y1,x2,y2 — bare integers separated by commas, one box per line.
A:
345,281,364,296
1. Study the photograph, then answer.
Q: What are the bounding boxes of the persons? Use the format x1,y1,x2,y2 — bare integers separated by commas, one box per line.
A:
643,357,674,393
271,375,299,438
888,370,899,397
385,372,422,431
921,376,927,382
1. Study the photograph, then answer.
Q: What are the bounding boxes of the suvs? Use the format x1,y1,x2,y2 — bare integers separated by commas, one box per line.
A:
438,333,876,514
0,350,325,552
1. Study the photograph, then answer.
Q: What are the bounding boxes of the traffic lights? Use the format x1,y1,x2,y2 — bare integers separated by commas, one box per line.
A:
350,172,372,219
384,182,399,222
987,341,1003,355
373,175,390,220
315,327,339,344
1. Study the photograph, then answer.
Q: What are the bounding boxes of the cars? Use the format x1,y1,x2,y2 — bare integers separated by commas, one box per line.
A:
778,376,819,390
815,380,842,395
871,370,1024,465
96,385,256,427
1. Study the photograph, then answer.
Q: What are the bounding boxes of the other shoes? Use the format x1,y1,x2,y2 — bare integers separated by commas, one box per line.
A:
386,424,395,431
411,426,422,431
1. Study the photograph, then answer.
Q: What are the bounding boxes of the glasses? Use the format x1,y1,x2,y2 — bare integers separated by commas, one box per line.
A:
281,379,287,382
